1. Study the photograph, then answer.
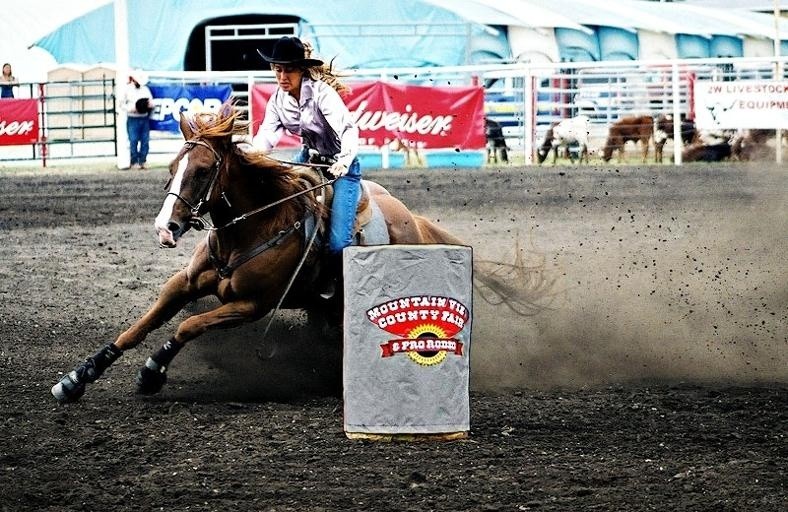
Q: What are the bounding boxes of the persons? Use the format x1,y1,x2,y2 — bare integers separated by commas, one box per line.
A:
0,62,21,98
243,32,365,311
125,67,157,171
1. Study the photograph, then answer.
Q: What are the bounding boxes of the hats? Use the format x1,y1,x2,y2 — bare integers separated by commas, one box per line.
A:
256,36,324,67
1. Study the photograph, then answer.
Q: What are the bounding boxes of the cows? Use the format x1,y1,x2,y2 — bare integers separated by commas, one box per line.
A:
654,113,776,162
601,112,657,164
484,117,511,167
535,112,594,167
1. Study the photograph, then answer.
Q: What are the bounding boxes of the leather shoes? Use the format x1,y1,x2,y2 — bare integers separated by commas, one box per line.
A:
317,274,336,300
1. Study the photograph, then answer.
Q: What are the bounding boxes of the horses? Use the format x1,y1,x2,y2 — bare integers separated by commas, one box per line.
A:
50,94,577,404
